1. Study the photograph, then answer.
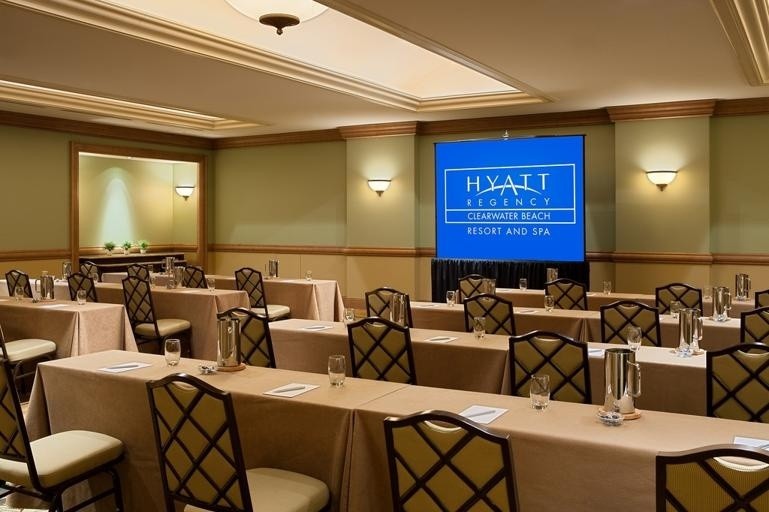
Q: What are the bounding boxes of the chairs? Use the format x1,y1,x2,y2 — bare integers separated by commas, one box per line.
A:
145,373,331,512
706,344,767,421
5,269,32,298
599,301,660,346
234,267,291,321
1,332,57,389
384,410,520,512
457,274,495,304
347,316,415,385
216,307,276,366
366,287,414,328
182,266,207,289
754,290,767,310
655,282,704,316
127,263,153,284
464,293,517,337
1,346,125,511
545,278,588,310
121,275,192,358
654,443,769,511
740,309,768,344
80,261,102,282
66,272,98,302
508,328,592,407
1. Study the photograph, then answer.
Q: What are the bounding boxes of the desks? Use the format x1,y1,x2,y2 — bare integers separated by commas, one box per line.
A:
102,271,346,321
458,288,758,319
2,279,250,360
268,319,708,416
409,302,740,352
1,295,137,357
65,350,768,510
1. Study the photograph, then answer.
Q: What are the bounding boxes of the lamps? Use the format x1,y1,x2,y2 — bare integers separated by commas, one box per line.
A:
225,0,329,35
645,170,678,191
175,186,194,201
368,180,392,198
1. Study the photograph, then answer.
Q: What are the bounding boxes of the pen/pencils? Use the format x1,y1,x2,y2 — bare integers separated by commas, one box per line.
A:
274,386,305,393
466,410,495,418
588,350,602,352
308,327,324,329
759,444,769,449
107,365,138,369
431,338,449,341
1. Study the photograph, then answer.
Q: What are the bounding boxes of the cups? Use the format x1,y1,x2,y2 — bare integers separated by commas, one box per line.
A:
627,326,645,351
265,260,279,278
343,267,560,342
603,347,643,415
529,373,551,411
216,316,242,366
327,355,346,388
12,260,98,305
670,271,753,358
148,257,217,292
305,270,313,281
604,281,611,294
164,339,181,365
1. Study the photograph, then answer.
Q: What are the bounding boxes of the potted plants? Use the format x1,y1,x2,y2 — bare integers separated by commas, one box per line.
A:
103,241,116,256
121,240,133,255
135,240,151,255
